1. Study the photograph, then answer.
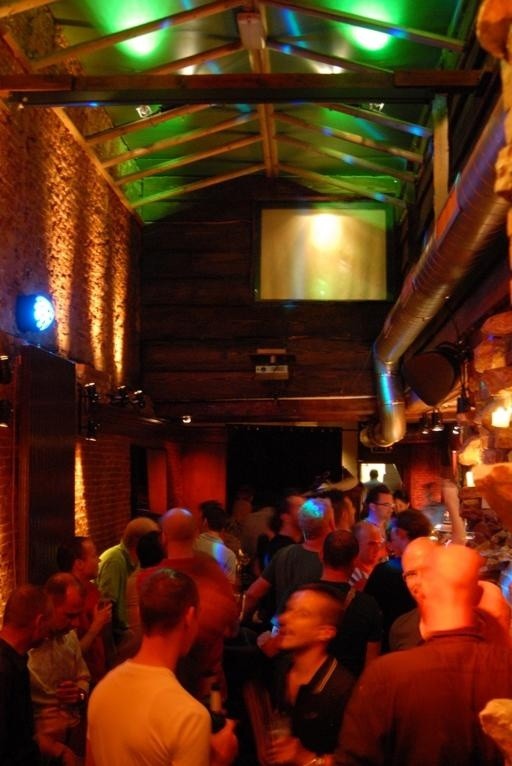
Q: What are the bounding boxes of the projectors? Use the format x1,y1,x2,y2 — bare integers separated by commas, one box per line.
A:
255,365,289,380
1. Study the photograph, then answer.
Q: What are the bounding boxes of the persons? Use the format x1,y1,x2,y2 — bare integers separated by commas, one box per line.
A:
3,481,512,766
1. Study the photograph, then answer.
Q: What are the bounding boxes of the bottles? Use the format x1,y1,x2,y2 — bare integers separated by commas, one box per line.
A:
208,684,225,733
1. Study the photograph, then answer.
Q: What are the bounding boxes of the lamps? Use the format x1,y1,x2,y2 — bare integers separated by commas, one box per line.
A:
79,380,149,408
14,291,57,335
75,417,103,443
